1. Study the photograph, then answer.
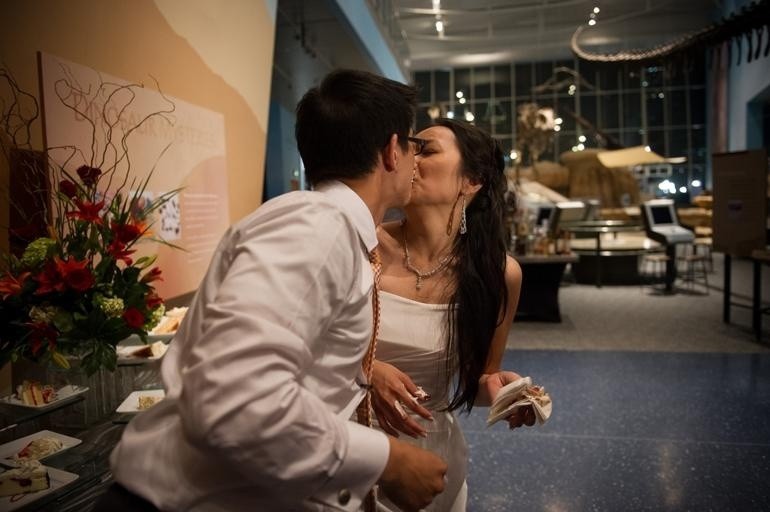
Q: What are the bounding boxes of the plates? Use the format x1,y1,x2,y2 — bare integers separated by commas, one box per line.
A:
112,314,182,366
115,389,167,414
0,381,91,512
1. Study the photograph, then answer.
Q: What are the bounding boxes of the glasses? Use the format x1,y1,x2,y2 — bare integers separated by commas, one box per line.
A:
381,134,424,156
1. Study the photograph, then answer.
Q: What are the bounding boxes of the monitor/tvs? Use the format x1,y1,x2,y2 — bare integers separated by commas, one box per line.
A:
646,200,679,228
536,205,551,226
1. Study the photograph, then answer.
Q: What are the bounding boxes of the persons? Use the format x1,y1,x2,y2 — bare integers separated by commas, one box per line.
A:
364,116,536,511
91,64,450,512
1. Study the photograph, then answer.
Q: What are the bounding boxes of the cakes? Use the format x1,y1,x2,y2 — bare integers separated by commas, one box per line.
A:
17,378,57,406
138,396,163,409
0,460,50,497
11,437,62,463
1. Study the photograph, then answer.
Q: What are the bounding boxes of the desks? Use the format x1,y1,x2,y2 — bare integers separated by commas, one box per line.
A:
512,250,578,324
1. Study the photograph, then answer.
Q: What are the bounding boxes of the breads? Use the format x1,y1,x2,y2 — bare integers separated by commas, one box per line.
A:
127,340,167,358
154,317,180,334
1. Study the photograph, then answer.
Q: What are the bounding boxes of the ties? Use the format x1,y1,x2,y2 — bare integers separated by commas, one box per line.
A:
357,245,381,429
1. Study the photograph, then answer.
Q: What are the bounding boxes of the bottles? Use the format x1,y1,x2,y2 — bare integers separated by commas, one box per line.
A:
534,230,571,255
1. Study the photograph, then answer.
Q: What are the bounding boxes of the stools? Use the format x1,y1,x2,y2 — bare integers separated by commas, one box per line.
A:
640,227,714,295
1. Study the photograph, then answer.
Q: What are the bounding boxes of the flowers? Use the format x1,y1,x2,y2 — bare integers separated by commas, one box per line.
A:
0,164,189,382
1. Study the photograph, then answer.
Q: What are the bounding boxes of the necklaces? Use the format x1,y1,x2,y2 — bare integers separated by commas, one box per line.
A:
399,218,455,291
